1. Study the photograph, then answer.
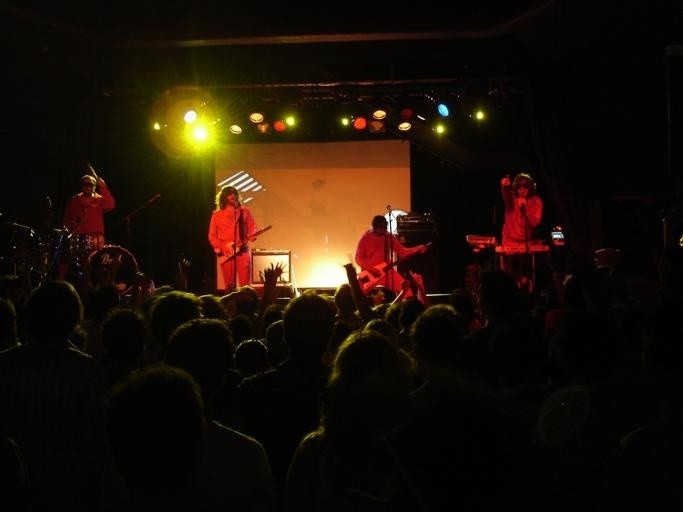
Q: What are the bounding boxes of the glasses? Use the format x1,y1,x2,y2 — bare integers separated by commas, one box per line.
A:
517,184,528,188
83,183,95,186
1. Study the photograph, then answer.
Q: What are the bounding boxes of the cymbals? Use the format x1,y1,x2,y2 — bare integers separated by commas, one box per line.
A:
0,223,34,237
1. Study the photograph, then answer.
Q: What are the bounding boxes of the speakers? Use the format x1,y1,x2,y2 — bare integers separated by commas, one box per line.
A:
395,230,438,295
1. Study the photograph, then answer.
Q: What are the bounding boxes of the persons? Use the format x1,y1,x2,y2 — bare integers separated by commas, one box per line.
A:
501,174,543,246
3,256,682,511
208,187,257,289
355,216,428,300
64,175,115,233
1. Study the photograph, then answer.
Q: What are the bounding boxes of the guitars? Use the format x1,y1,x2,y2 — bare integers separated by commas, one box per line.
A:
217,223,272,265
358,242,432,291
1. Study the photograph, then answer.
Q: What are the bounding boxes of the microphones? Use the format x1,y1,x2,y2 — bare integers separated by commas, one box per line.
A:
386,204,393,214
519,203,526,214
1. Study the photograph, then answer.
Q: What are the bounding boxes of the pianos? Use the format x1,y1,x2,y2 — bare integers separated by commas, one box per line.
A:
473,245,550,253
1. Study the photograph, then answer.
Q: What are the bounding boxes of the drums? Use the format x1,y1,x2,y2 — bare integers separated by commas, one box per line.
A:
87,245,138,282
46,228,69,252
